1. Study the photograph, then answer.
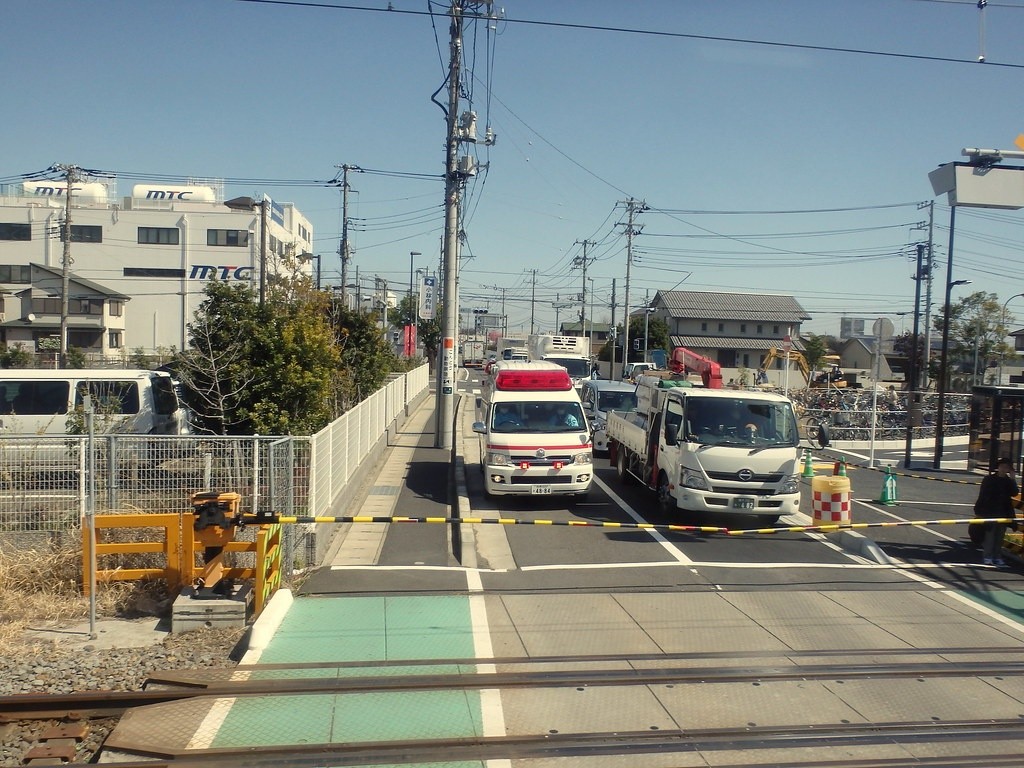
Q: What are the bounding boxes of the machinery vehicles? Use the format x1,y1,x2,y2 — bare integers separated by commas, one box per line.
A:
757,346,847,388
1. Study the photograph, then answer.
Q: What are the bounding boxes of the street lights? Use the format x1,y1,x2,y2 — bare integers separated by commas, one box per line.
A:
933,205,973,469
999,294,1024,386
644,307,659,363
296,253,321,290
224,197,268,305
409,252,422,358
345,284,360,321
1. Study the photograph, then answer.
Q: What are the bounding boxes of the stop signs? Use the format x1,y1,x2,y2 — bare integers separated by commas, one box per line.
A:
783,335,792,352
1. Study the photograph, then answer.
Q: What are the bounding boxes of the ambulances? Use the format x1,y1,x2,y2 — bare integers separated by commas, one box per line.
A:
472,359,600,499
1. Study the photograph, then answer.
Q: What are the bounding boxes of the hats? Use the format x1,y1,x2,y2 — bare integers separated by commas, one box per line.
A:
996,458,1013,468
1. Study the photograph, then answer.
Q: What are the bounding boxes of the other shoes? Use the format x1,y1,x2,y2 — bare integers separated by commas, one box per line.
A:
983,557,994,565
993,558,1005,565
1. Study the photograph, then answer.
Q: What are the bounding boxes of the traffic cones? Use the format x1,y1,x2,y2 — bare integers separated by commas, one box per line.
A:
881,467,898,506
801,449,815,478
838,456,847,476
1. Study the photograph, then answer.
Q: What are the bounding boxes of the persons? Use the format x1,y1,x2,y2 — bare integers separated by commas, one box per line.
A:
688,402,742,435
726,378,734,387
551,404,579,428
974,458,1019,565
495,405,524,428
886,385,898,416
591,363,601,380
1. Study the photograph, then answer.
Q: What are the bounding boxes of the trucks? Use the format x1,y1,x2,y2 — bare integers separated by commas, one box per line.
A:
462,341,483,367
624,363,658,384
483,335,600,397
606,387,830,524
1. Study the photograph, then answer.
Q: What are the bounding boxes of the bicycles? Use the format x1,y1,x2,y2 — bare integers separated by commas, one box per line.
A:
787,389,938,438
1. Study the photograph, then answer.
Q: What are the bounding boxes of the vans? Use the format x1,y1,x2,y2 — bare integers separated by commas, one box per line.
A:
580,380,636,451
1,370,199,472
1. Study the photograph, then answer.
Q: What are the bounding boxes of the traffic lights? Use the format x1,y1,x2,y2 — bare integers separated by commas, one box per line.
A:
610,327,616,341
633,339,639,349
474,309,488,314
